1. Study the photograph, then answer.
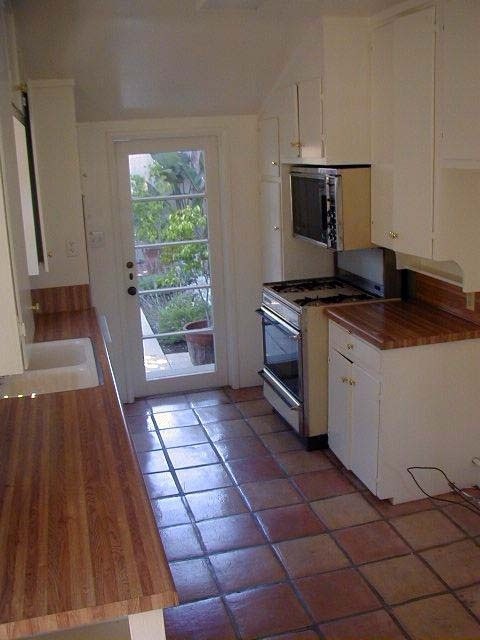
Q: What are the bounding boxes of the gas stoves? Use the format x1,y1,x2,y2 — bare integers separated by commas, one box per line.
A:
261,276,379,313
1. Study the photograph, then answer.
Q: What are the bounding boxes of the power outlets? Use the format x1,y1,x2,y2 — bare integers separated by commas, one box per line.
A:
64,241,80,260
90,232,104,249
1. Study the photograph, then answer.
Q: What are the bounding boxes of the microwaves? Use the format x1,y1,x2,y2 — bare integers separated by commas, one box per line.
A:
289,167,373,251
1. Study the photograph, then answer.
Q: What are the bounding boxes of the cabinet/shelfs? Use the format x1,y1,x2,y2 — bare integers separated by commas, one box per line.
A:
275,78,326,162
26,78,91,290
370,0,480,293
328,319,480,505
259,120,284,285
8,114,54,276
0,0,39,378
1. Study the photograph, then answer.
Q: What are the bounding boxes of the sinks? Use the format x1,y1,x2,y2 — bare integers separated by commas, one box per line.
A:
0,373,80,396
26,342,89,373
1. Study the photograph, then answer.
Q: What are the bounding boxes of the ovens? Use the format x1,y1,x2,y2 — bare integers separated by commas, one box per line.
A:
253,304,327,439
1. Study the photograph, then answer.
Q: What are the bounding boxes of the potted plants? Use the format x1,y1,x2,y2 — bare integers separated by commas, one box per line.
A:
130,176,167,275
156,204,214,366
